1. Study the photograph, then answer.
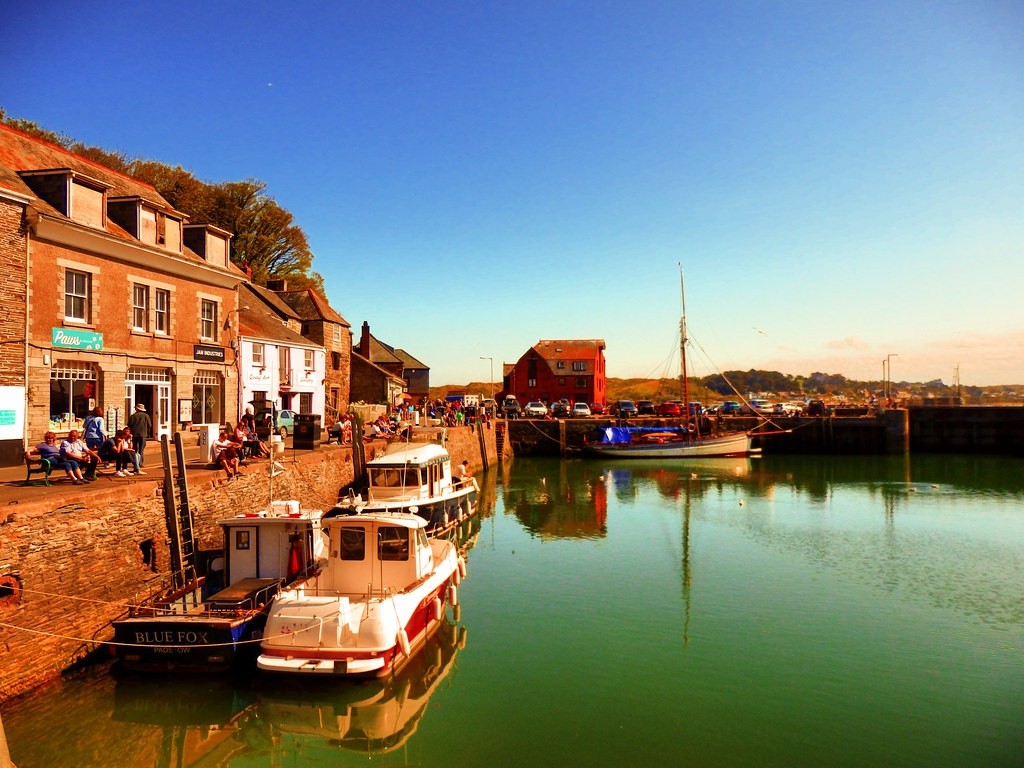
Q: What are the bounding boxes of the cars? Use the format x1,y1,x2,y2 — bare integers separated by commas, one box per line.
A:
482,399,498,413
739,399,774,416
572,403,590,417
706,403,722,414
589,402,605,415
656,398,702,418
248,399,297,439
722,401,740,414
776,403,803,415
524,401,547,419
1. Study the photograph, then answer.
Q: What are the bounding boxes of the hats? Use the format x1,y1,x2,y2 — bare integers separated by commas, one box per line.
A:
136,404,146,411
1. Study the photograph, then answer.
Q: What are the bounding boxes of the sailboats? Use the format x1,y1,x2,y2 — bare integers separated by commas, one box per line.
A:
583,261,793,461
591,453,764,655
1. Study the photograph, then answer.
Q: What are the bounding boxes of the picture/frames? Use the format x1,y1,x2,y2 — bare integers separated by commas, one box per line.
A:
178,399,193,422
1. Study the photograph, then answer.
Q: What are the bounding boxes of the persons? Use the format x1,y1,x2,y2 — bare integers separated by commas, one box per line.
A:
368,406,398,439
25,431,91,484
453,460,470,491
430,409,436,419
332,413,373,445
121,425,147,475
60,430,102,481
501,404,519,420
82,406,114,469
97,429,134,478
212,408,276,479
443,410,462,427
127,404,152,468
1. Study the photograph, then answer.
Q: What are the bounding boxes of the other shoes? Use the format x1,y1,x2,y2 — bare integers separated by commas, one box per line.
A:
81,478,90,484
134,471,147,474
73,479,84,485
86,477,95,481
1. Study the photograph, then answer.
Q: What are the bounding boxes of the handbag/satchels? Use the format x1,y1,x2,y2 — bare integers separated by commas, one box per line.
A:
82,428,87,439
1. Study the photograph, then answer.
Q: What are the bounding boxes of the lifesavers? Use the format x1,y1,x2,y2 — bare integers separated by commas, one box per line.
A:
688,423,695,432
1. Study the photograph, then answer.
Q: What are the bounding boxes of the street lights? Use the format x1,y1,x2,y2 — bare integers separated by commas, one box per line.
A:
480,357,493,399
882,353,899,401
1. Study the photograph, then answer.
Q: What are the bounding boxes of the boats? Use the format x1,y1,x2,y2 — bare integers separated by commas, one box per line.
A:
436,511,485,561
255,505,467,679
110,360,331,683
255,615,468,755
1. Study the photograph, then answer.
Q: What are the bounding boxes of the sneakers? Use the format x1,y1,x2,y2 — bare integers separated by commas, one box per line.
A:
227,472,234,478
233,471,246,476
114,471,126,477
122,469,134,476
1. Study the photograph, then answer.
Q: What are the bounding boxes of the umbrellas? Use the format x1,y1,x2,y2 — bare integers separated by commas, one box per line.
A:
395,393,412,404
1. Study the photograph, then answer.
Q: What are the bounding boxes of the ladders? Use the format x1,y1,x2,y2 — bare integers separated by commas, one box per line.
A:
351,418,367,479
478,422,489,473
161,433,197,584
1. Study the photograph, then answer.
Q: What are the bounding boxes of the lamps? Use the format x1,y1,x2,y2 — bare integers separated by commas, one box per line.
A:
227,306,249,326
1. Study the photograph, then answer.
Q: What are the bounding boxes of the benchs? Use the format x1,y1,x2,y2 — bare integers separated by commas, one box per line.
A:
82,435,143,472
206,577,288,619
24,452,100,486
325,426,340,444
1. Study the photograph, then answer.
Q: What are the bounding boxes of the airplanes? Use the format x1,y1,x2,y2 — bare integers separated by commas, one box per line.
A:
334,443,480,548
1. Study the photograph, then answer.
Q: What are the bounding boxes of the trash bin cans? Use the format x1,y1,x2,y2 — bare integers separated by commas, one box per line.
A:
293,413,321,450
465,407,477,423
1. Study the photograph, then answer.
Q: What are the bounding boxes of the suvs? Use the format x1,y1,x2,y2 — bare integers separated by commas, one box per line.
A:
551,399,571,418
636,400,654,415
806,399,825,416
501,395,521,418
615,399,638,418
